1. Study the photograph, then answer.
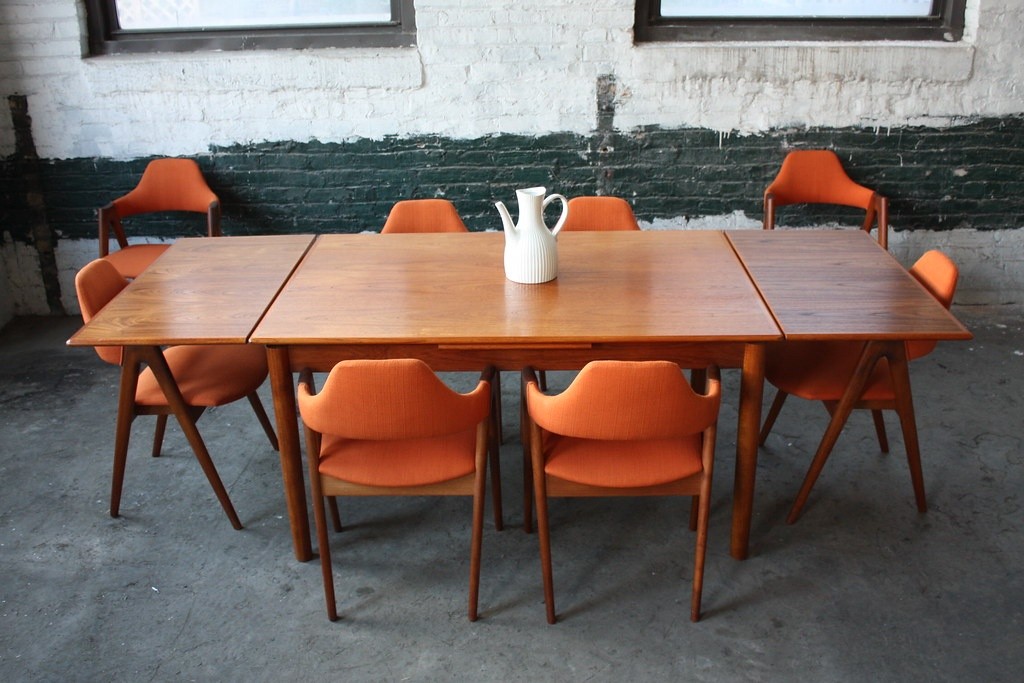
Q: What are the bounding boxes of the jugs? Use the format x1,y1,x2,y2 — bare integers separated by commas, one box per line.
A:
494,186,569,285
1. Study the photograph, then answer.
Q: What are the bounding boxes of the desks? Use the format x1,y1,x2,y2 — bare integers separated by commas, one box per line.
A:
63,228,974,564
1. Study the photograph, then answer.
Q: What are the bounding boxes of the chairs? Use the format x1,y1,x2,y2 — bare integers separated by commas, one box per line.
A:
74,256,282,531
760,148,889,248
520,355,722,623
95,159,221,279
295,357,504,623
759,247,959,526
